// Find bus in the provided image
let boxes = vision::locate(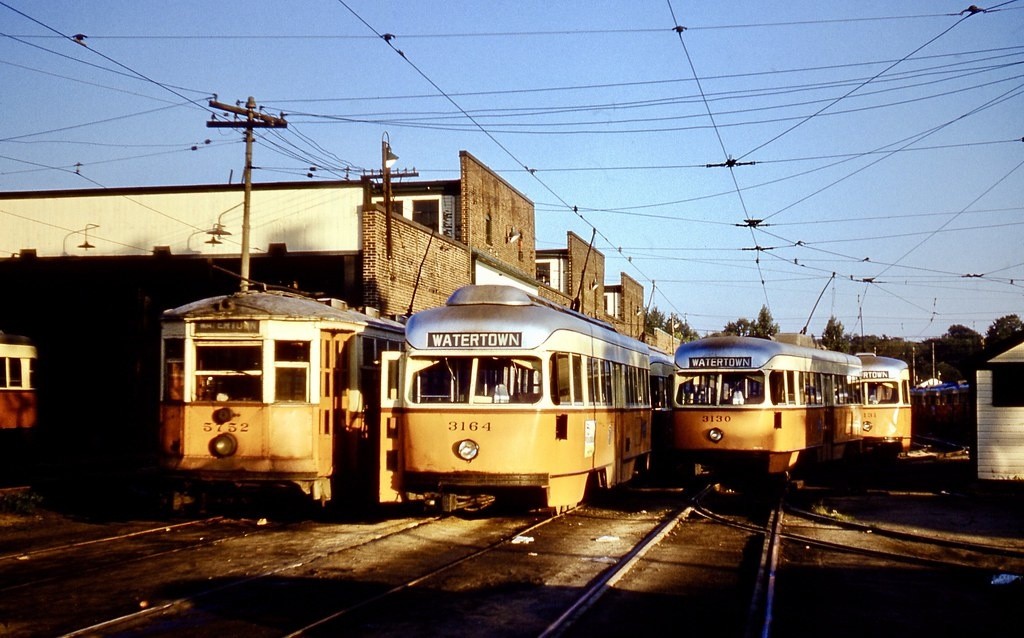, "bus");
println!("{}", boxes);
[674,336,970,492]
[159,288,406,510]
[0,332,38,453]
[402,283,676,515]
